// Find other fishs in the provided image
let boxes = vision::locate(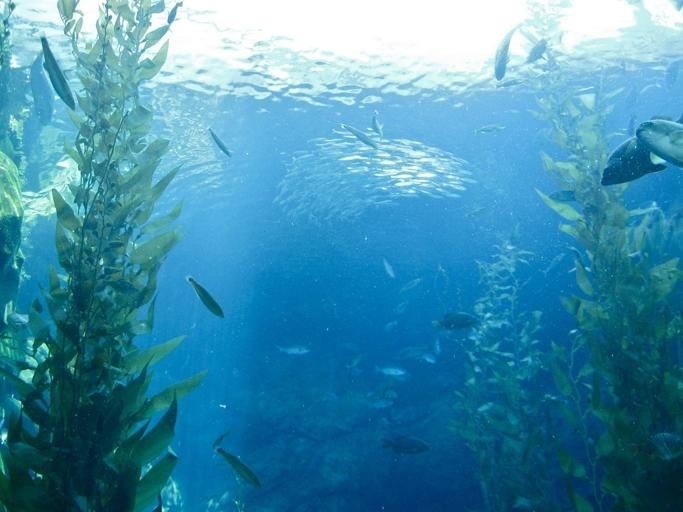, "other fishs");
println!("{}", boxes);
[275,344,312,355]
[41,36,76,111]
[167,1,182,24]
[542,253,567,278]
[345,254,494,453]
[474,124,505,136]
[29,51,55,127]
[207,127,232,159]
[186,275,224,318]
[548,191,576,200]
[650,432,683,459]
[496,79,525,88]
[273,108,483,226]
[524,39,547,64]
[601,112,683,185]
[495,23,522,80]
[213,431,262,490]
[635,119,683,170]
